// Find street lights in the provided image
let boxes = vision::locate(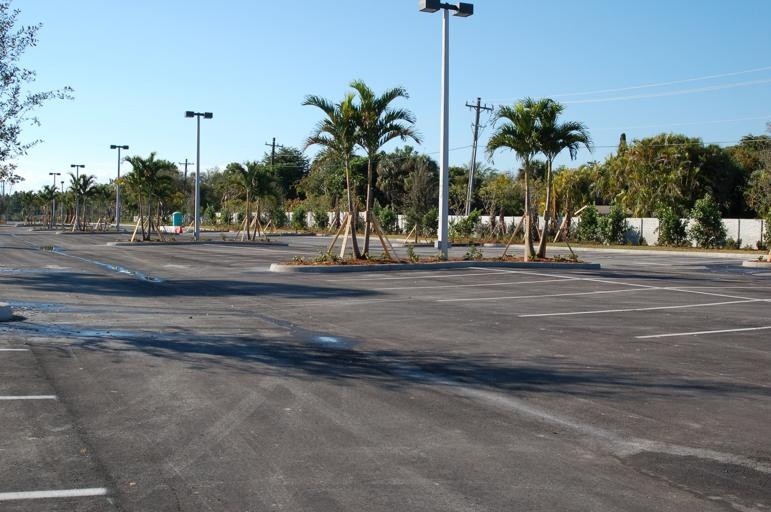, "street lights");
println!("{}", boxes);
[420,0,475,260]
[110,143,129,233]
[47,171,62,227]
[70,164,86,230]
[185,112,217,240]
